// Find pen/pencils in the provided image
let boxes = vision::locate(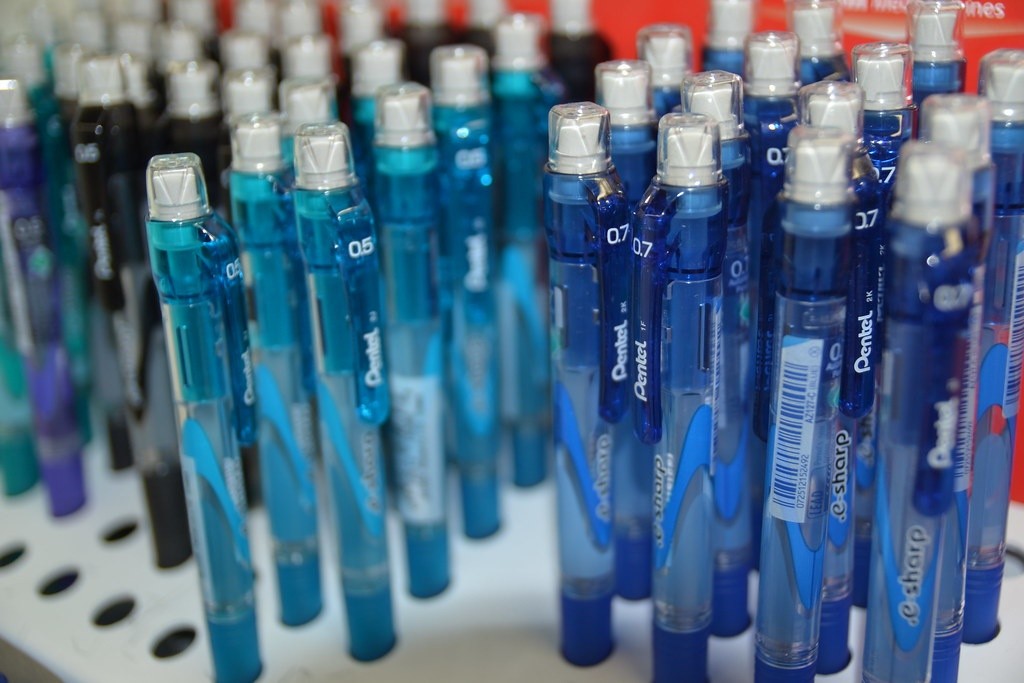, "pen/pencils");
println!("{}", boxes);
[0,0,1024,683]
